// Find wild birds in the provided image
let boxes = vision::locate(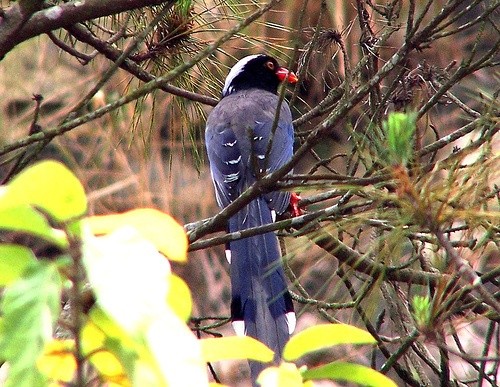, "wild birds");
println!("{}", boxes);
[203,52,308,387]
